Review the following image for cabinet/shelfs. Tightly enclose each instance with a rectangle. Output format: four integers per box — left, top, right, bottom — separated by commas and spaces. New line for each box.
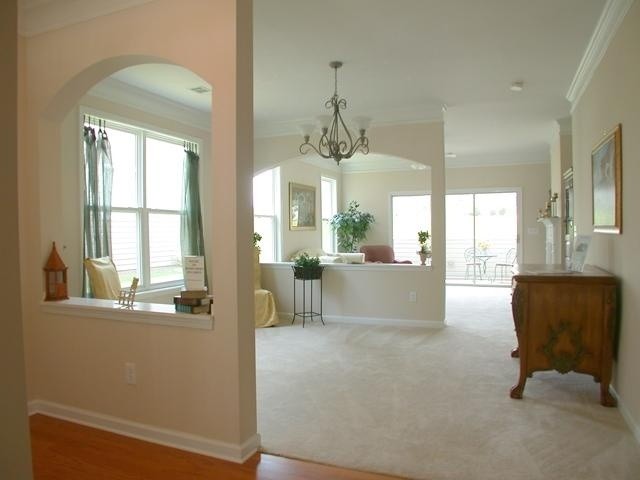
505, 264, 622, 410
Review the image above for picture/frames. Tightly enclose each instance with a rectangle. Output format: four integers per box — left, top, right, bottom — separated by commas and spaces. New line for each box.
287, 180, 317, 231
590, 123, 624, 236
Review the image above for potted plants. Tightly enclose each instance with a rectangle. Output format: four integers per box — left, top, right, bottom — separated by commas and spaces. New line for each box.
415, 230, 431, 265
291, 252, 325, 279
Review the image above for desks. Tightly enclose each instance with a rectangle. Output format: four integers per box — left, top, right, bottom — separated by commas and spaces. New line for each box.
472, 255, 495, 280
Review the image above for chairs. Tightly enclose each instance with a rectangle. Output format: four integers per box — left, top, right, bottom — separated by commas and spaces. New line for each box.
463, 247, 483, 281
361, 244, 413, 264
491, 248, 518, 281
253, 247, 280, 329
85, 254, 123, 300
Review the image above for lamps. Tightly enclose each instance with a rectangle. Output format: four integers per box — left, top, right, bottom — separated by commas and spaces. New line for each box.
297, 57, 370, 167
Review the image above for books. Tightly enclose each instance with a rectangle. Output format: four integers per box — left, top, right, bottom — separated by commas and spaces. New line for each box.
173, 256, 210, 314
